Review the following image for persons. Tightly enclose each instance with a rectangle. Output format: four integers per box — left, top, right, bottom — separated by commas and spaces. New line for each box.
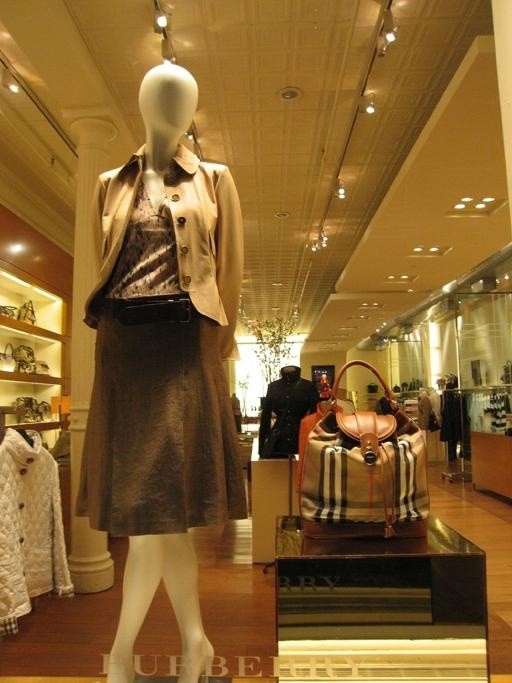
440, 376, 468, 466
259, 365, 320, 457
73, 60, 249, 683
230, 393, 243, 433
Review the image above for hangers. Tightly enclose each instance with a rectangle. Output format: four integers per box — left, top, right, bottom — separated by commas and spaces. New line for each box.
0, 424, 59, 469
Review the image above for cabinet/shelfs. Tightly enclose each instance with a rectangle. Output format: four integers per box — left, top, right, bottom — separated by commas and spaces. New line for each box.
0, 258, 71, 454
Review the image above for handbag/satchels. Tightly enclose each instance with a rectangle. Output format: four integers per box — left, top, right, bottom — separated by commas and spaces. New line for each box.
0, 343, 49, 375
295, 360, 430, 540
18, 300, 36, 325
15, 397, 51, 424
0, 306, 17, 317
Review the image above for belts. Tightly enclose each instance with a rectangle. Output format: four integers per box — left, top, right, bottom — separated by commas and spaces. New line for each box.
113, 294, 197, 326
